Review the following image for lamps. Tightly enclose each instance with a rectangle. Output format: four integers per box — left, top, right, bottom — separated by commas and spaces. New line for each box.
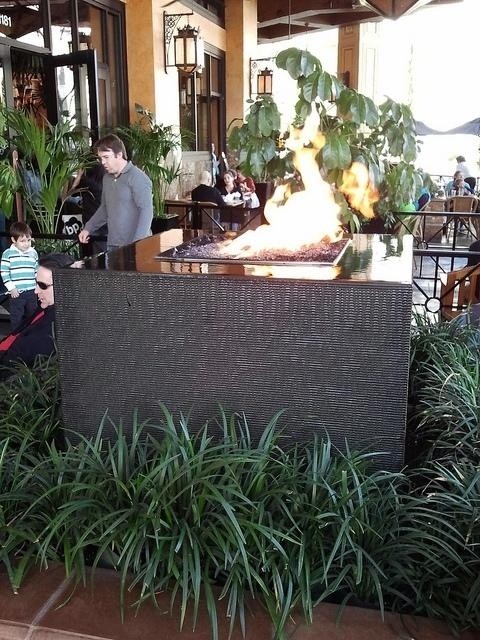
186, 75, 203, 110
249, 58, 277, 98
163, 9, 205, 74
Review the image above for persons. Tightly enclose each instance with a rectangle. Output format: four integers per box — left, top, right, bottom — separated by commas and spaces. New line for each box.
0, 222, 40, 333
187, 165, 260, 230
0, 254, 73, 383
68, 132, 132, 255
77, 135, 153, 269
467, 241, 480, 301
410, 156, 480, 211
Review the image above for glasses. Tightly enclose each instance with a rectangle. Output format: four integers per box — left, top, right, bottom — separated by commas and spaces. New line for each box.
35, 278, 53, 289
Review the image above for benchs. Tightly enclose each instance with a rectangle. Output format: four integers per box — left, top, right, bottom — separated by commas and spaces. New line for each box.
0, 559, 478, 640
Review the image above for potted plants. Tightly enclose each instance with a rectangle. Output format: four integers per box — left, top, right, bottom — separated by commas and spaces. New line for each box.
94, 103, 197, 235
